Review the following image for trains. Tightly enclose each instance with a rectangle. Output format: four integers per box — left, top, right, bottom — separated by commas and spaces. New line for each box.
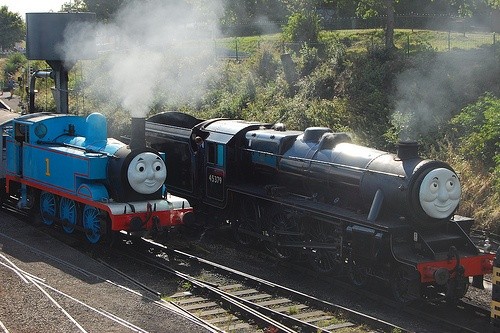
1, 112, 193, 246
118, 111, 494, 306
